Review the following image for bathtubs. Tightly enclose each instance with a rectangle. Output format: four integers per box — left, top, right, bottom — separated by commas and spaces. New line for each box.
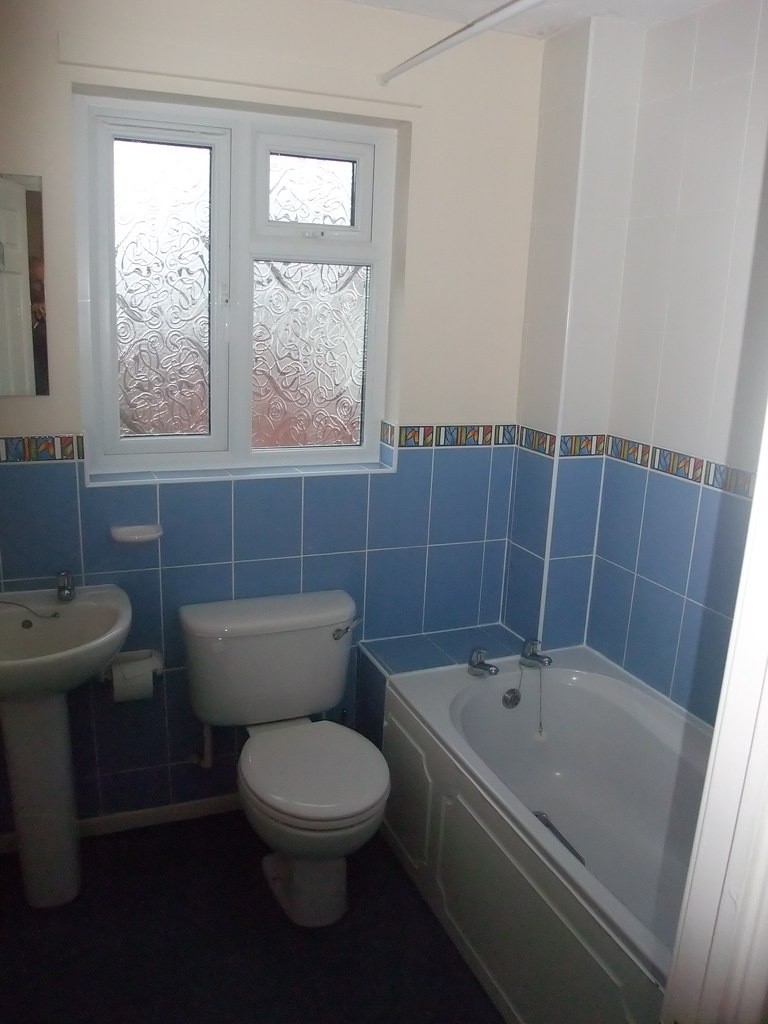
381, 644, 718, 1023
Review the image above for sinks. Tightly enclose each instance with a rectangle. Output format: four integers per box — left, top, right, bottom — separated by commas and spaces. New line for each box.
0, 584, 130, 701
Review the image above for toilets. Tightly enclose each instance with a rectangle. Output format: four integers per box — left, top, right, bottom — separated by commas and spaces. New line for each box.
180, 592, 391, 928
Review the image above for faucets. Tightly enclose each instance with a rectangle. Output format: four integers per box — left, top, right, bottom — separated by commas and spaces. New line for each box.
57, 560, 78, 601
522, 642, 553, 667
469, 648, 499, 678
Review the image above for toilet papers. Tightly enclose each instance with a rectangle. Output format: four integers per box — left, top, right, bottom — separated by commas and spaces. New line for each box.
111, 661, 155, 702
